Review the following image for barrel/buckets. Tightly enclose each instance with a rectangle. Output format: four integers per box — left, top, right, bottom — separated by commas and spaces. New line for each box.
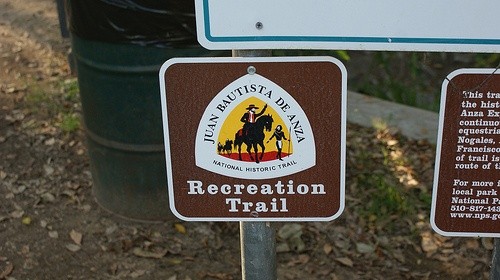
67, 0, 234, 225
